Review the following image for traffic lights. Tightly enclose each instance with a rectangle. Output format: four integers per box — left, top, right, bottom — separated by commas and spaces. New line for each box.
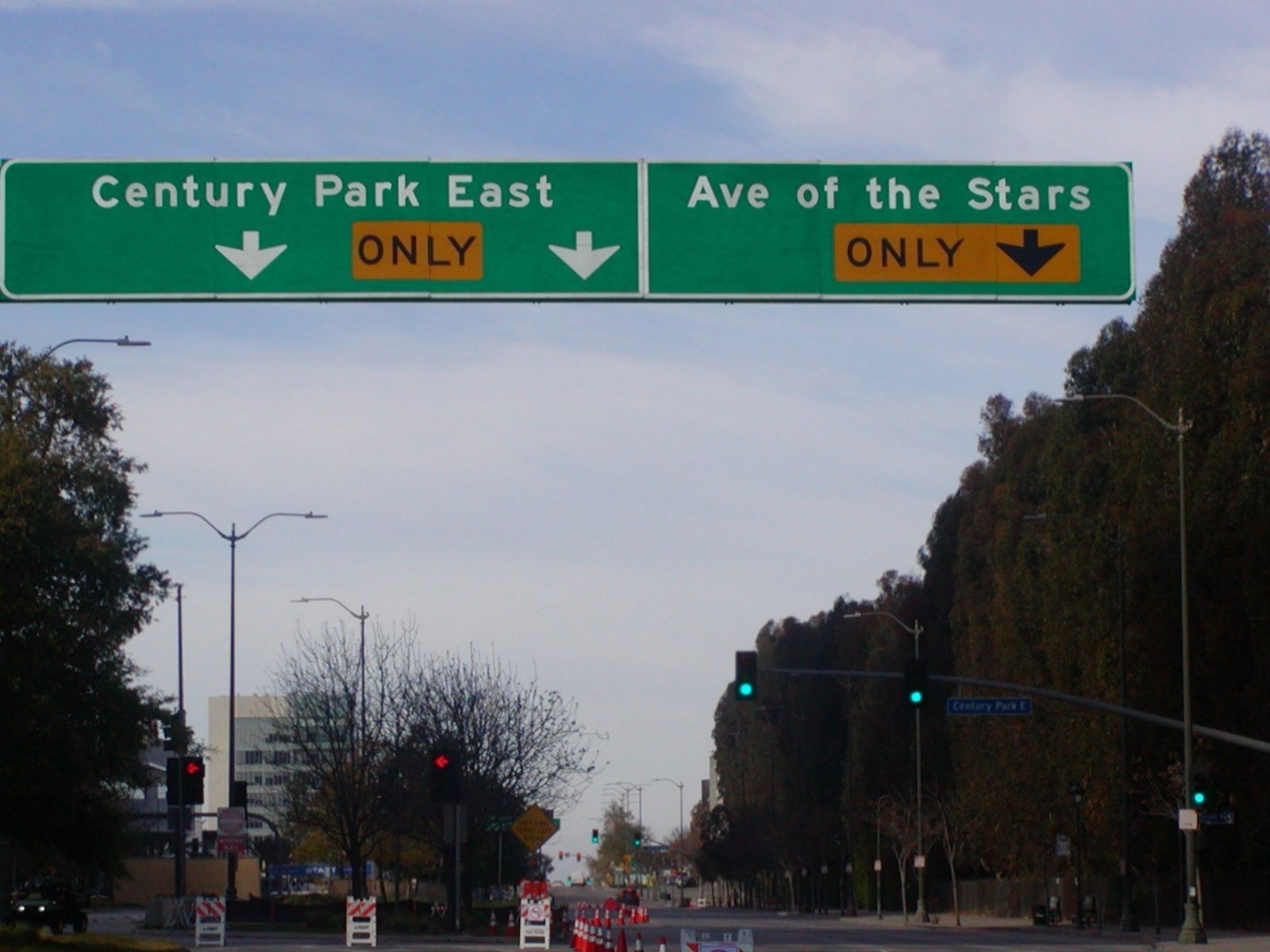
559, 851, 563, 860
592, 829, 600, 843
1190, 780, 1205, 814
167, 753, 206, 804
911, 661, 929, 713
735, 652, 760, 702
578, 853, 582, 861
232, 781, 248, 818
552, 819, 560, 829
634, 832, 643, 846
567, 876, 571, 885
428, 747, 461, 781
161, 708, 188, 751
586, 876, 592, 885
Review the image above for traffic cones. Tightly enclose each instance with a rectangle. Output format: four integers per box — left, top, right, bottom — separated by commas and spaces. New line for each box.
549, 912, 554, 934
508, 912, 518, 938
489, 911, 499, 937
562, 899, 667, 952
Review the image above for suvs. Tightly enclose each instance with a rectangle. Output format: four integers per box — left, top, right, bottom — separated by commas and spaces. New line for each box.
616, 889, 641, 905
675, 875, 699, 888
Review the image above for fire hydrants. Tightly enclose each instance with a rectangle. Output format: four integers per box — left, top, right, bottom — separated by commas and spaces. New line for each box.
931, 914, 940, 924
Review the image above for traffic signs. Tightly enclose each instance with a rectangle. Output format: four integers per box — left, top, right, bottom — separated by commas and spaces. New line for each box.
2, 159, 639, 301
641, 162, 1133, 303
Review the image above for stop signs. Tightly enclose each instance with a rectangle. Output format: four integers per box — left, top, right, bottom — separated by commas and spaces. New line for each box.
219, 807, 246, 836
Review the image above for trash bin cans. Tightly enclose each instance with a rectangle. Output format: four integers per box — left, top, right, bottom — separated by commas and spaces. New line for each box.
1031, 905, 1046, 925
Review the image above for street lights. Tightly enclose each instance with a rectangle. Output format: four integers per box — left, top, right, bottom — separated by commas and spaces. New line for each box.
848, 611, 930, 922
300, 597, 370, 896
1054, 390, 1207, 944
139, 509, 329, 923
592, 782, 643, 895
650, 778, 684, 906
